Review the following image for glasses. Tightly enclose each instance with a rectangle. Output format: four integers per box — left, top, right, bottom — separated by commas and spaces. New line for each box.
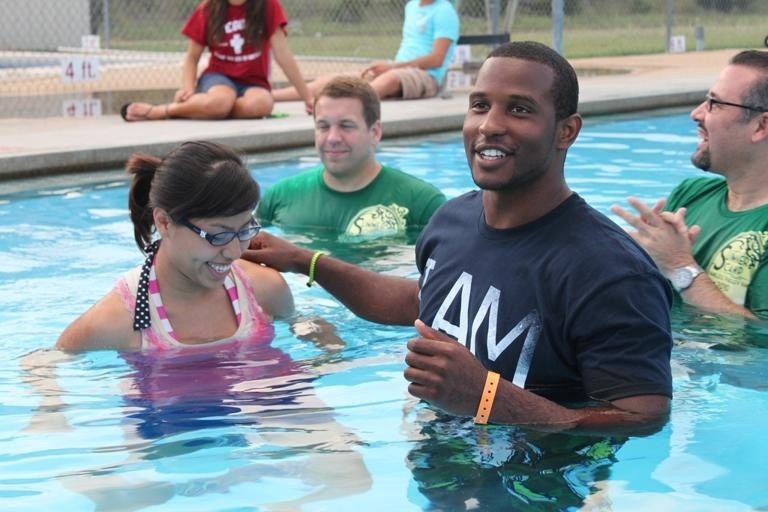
172, 217, 261, 248
704, 95, 759, 116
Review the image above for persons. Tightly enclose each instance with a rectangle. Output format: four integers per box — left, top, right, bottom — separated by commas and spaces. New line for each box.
608, 49, 767, 318
21, 140, 370, 510
251, 76, 448, 237
120, 0, 315, 120
243, 40, 673, 436
270, 0, 460, 105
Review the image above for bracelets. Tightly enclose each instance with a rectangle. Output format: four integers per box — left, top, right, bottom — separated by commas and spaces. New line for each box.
305, 251, 326, 287
472, 371, 502, 427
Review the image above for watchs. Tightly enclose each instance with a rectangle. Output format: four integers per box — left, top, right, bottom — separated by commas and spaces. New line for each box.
671, 263, 705, 293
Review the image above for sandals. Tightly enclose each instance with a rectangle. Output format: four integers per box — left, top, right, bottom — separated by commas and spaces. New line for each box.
120, 102, 170, 121
